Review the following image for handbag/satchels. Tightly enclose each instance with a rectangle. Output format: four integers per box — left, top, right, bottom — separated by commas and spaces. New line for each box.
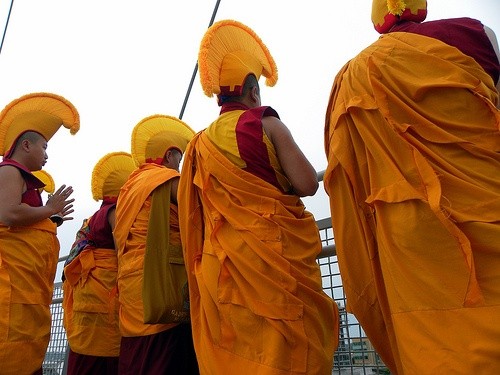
143, 180, 191, 325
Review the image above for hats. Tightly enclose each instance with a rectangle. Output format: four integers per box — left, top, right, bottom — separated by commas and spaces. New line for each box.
0, 92, 80, 159
91, 151, 139, 202
198, 19, 278, 97
31, 170, 55, 194
131, 114, 197, 167
370, 0, 427, 33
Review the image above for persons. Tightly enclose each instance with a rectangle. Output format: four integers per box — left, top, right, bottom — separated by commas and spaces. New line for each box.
324, 0, 500, 375
0, 92, 81, 375
60, 19, 339, 375
